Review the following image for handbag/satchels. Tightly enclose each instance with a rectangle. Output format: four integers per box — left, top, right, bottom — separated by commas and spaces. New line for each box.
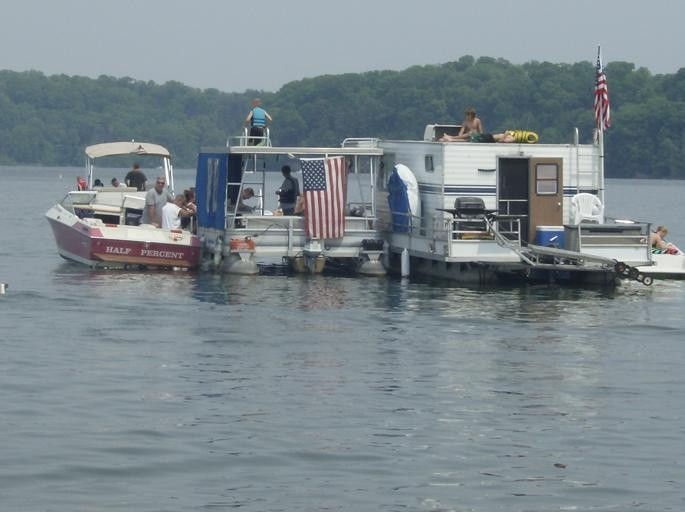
277, 177, 298, 204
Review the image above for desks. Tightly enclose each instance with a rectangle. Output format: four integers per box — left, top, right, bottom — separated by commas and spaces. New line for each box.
435, 203, 507, 239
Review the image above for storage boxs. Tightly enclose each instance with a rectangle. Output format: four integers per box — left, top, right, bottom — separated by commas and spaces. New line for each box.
534, 225, 564, 249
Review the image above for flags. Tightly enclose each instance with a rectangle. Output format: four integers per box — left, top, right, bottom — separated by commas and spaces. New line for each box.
594, 56, 611, 132
299, 156, 344, 240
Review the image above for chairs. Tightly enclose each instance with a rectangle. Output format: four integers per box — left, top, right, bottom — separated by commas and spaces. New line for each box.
570, 192, 605, 226
451, 197, 488, 238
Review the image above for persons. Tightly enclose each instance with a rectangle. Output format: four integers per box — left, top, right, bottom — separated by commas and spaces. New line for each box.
245, 98, 272, 146
276, 165, 299, 216
458, 109, 482, 141
77, 163, 197, 232
238, 187, 255, 212
442, 132, 518, 143
651, 226, 668, 250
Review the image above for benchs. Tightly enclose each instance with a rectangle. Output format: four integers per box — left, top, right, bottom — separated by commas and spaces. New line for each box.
91, 186, 139, 209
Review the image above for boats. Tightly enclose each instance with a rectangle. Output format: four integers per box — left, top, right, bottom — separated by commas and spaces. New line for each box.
44, 138, 201, 272
